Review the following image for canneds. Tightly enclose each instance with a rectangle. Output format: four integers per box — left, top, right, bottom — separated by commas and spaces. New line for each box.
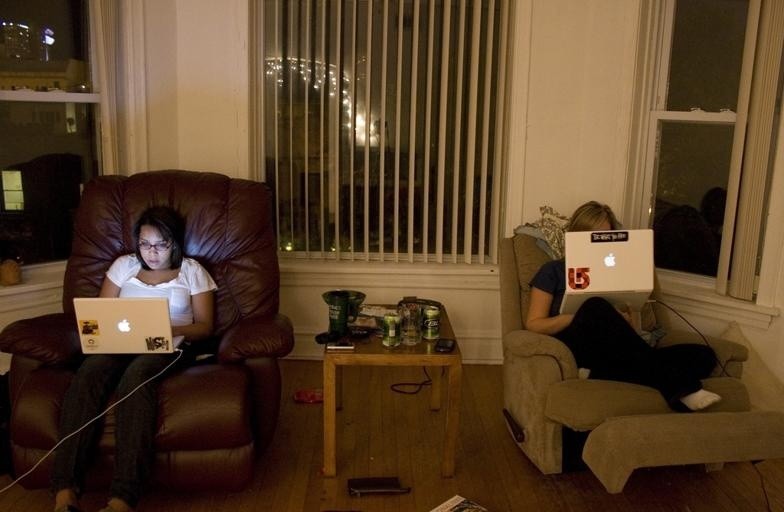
423, 306, 440, 339
382, 312, 401, 347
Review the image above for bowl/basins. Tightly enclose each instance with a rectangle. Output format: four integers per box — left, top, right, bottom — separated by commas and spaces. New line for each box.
323, 290, 366, 306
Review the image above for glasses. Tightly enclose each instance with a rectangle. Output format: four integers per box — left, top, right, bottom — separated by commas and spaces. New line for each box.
138, 240, 172, 251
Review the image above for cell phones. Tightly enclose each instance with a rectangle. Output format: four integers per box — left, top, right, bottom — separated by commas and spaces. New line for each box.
435, 338, 457, 353
326, 341, 356, 349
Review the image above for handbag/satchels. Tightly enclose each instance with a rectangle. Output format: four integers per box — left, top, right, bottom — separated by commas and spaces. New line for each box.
349, 477, 411, 497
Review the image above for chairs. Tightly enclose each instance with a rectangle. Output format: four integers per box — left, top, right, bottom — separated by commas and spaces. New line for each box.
498, 233, 783, 489
1, 169, 295, 489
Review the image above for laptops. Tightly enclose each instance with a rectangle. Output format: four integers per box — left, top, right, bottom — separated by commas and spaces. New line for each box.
560, 230, 656, 314
71, 297, 184, 357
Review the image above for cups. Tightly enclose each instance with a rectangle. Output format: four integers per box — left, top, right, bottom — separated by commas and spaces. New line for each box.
398, 303, 422, 345
328, 291, 357, 333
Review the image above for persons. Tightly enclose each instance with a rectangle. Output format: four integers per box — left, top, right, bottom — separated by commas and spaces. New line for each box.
526, 200, 721, 413
51, 204, 218, 511
